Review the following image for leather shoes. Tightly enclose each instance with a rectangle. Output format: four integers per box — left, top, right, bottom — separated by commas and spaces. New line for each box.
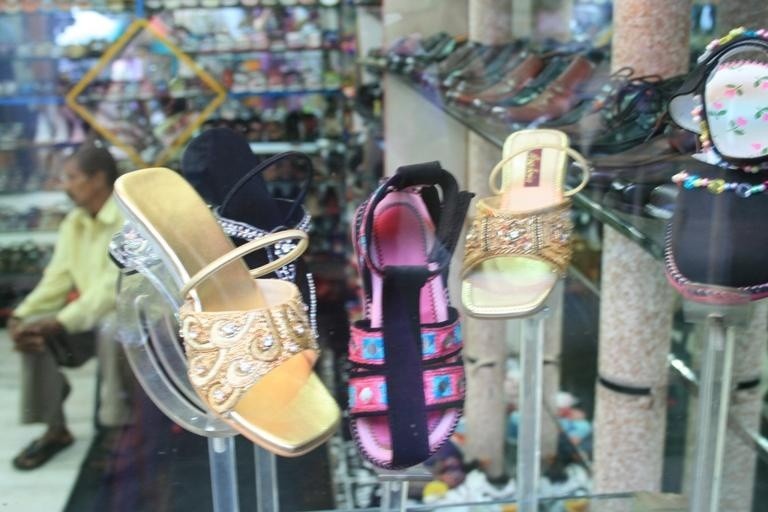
473, 37, 707, 221
365, 29, 480, 106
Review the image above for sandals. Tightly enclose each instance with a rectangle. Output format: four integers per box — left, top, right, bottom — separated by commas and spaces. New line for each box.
181, 127, 319, 336
460, 129, 590, 319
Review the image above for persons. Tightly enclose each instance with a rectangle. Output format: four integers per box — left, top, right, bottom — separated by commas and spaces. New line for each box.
5, 142, 180, 469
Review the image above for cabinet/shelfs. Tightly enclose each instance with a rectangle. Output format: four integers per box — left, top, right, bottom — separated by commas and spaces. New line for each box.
0, 0, 359, 329
385, 32, 768, 512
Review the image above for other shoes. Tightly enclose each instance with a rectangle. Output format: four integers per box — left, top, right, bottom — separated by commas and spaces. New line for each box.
0, 0, 360, 161
99, 424, 142, 475
664, 28, 768, 305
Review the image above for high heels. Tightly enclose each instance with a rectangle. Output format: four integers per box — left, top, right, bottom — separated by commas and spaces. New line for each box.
107, 166, 345, 456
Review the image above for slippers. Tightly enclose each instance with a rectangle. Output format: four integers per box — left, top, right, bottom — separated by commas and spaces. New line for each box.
13, 434, 76, 471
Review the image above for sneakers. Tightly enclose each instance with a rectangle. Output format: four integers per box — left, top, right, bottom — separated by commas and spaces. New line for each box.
337, 361, 593, 511
